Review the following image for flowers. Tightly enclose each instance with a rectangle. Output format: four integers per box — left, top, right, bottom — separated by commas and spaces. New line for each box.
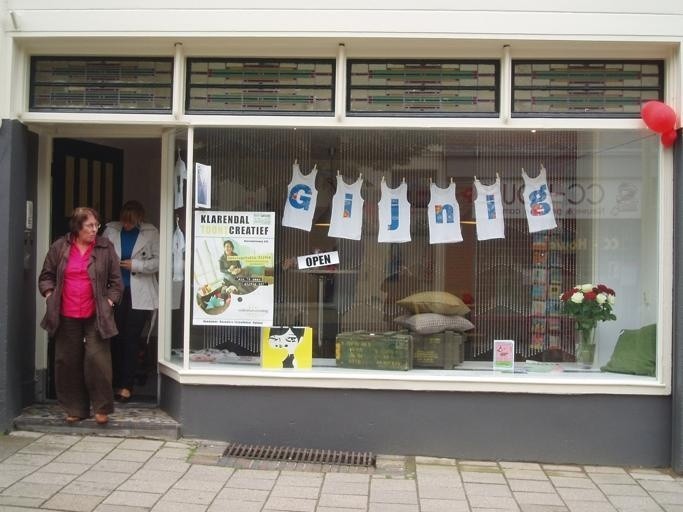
556, 283, 617, 364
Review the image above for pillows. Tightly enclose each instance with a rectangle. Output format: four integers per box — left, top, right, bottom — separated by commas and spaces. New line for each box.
393, 290, 475, 334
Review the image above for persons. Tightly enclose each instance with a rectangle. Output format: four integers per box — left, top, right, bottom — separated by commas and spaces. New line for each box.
219, 239, 241, 286
101, 199, 160, 401
39, 206, 125, 424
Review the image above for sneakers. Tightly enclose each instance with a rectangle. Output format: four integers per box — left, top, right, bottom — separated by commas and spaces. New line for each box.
115, 389, 131, 402
66, 416, 80, 421
96, 414, 107, 423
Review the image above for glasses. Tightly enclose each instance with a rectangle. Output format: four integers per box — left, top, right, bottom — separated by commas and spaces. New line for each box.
86, 223, 101, 228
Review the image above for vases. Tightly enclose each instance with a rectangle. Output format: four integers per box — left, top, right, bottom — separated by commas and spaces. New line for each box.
570, 319, 598, 370
